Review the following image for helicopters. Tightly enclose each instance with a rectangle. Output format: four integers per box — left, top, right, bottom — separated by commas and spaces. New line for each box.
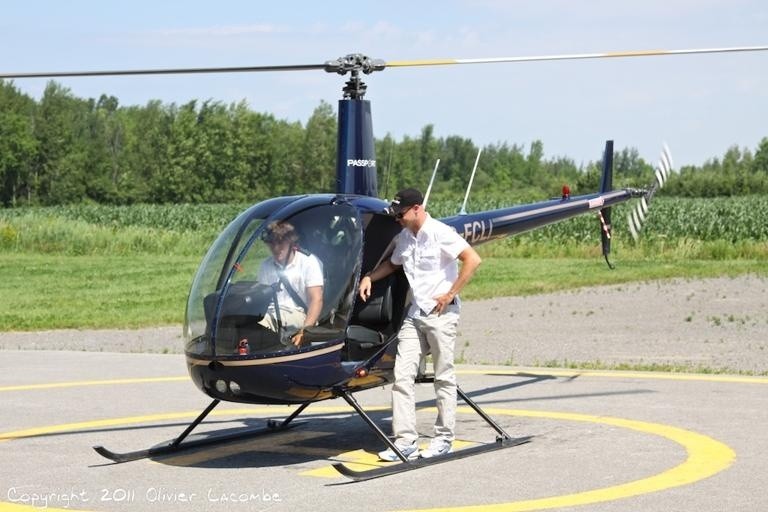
1, 43, 768, 488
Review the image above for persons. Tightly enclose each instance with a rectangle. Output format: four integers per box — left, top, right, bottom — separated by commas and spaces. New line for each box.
359, 189, 482, 462
256, 219, 325, 350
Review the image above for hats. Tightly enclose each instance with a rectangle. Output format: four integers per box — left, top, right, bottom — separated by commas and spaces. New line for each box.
391, 188, 424, 208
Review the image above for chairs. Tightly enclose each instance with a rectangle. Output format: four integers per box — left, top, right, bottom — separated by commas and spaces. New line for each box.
303, 262, 396, 359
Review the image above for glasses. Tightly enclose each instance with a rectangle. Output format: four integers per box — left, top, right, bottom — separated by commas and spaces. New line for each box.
393, 206, 413, 218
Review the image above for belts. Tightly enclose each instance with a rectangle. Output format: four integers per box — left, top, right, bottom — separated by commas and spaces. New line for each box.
448, 299, 456, 305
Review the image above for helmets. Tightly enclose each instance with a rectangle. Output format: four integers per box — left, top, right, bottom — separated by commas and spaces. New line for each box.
261, 219, 300, 242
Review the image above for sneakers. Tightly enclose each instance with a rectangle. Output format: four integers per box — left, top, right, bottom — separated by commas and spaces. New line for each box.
378, 439, 456, 462
